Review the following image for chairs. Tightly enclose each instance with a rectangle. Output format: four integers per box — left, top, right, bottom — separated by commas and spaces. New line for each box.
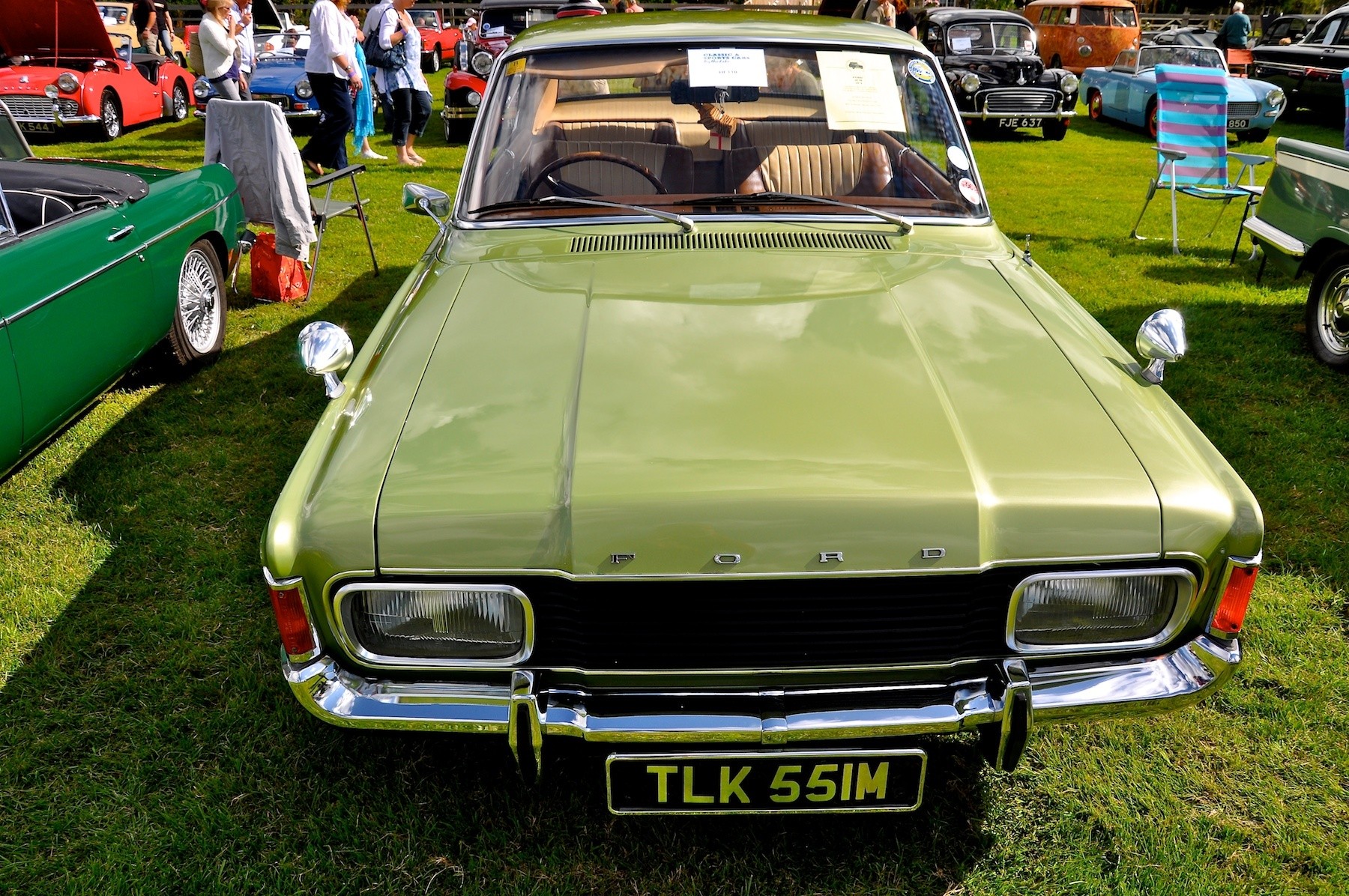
541, 121, 674, 145
731, 142, 896, 198
529, 140, 694, 194
1128, 63, 1272, 261
732, 120, 861, 144
203, 97, 380, 304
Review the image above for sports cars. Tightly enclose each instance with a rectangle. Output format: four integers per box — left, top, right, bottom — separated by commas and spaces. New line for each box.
189, 0, 385, 129
1077, 45, 1287, 142
0, 0, 198, 142
0, 94, 251, 490
403, 9, 468, 73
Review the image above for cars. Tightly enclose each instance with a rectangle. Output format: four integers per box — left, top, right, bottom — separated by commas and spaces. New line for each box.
1249, 3, 1349, 129
1139, 25, 1227, 69
439, 0, 610, 143
901, 9, 1082, 141
91, 2, 189, 72
1249, 14, 1326, 76
258, 10, 1269, 830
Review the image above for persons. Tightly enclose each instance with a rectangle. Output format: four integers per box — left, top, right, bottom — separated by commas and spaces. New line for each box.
1218, 2, 1252, 73
611, 0, 645, 14
416, 17, 427, 26
282, 28, 308, 56
466, 17, 477, 31
133, 0, 175, 61
852, 0, 917, 40
760, 55, 821, 96
556, 0, 610, 101
198, 0, 257, 101
297, 0, 433, 176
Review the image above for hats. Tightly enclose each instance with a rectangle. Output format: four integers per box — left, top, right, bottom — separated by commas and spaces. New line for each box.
466, 16, 478, 26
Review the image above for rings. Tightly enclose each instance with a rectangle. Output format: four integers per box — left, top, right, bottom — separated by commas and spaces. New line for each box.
356, 84, 359, 87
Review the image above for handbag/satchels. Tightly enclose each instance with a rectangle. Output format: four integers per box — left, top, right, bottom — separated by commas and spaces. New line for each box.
362, 6, 413, 70
253, 231, 308, 302
189, 30, 206, 75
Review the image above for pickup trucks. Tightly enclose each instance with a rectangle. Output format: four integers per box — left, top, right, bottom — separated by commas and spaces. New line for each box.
1242, 137, 1349, 371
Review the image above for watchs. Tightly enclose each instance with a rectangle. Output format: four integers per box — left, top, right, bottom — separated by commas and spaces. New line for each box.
345, 66, 351, 70
145, 27, 148, 31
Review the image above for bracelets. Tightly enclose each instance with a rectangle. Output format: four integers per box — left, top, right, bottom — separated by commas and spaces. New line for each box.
400, 29, 407, 36
238, 21, 246, 27
170, 31, 175, 34
347, 70, 356, 78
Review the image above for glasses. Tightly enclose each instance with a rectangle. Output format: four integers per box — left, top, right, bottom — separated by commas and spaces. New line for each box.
225, 6, 230, 10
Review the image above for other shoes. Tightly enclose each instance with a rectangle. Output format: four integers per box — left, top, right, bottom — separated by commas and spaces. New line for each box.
399, 159, 425, 168
301, 156, 324, 176
410, 155, 428, 165
362, 151, 388, 160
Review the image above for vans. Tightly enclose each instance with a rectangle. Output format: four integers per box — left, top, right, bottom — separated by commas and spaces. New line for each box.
1017, 0, 1142, 76
